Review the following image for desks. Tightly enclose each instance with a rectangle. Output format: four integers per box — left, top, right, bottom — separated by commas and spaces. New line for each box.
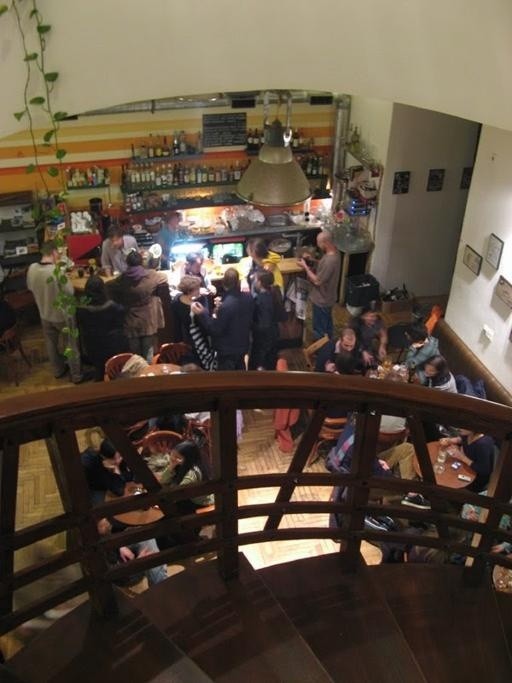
413, 439, 477, 489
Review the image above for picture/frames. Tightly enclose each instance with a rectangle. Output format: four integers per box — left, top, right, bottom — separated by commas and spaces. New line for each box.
485, 232, 505, 271
462, 243, 483, 276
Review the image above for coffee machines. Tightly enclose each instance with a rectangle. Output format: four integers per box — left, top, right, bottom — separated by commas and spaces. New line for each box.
89, 197, 106, 233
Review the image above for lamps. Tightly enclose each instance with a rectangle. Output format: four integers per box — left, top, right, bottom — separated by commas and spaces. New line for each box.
231, 89, 316, 209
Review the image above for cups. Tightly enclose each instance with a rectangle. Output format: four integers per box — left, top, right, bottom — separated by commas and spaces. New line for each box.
70, 211, 95, 234
179, 214, 226, 239
173, 184, 233, 205
369, 355, 408, 383
437, 448, 447, 463
127, 486, 143, 494
161, 194, 170, 206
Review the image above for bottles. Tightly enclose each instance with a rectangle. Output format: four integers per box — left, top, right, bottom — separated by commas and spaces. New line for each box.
122, 159, 252, 187
297, 155, 324, 175
246, 127, 316, 154
67, 165, 110, 187
346, 123, 361, 152
125, 191, 142, 211
324, 219, 353, 251
138, 124, 204, 159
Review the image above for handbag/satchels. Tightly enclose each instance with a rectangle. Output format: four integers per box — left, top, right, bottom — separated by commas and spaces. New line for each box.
189, 324, 212, 370
272, 305, 288, 322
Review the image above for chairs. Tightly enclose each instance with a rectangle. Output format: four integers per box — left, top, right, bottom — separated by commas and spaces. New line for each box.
1, 306, 33, 390
378, 427, 409, 451
307, 417, 350, 467
93, 337, 211, 533
388, 309, 443, 363
327, 467, 370, 543
303, 333, 331, 370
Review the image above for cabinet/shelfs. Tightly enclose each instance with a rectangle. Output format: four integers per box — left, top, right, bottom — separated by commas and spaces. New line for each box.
119, 142, 332, 247
0, 189, 44, 268
